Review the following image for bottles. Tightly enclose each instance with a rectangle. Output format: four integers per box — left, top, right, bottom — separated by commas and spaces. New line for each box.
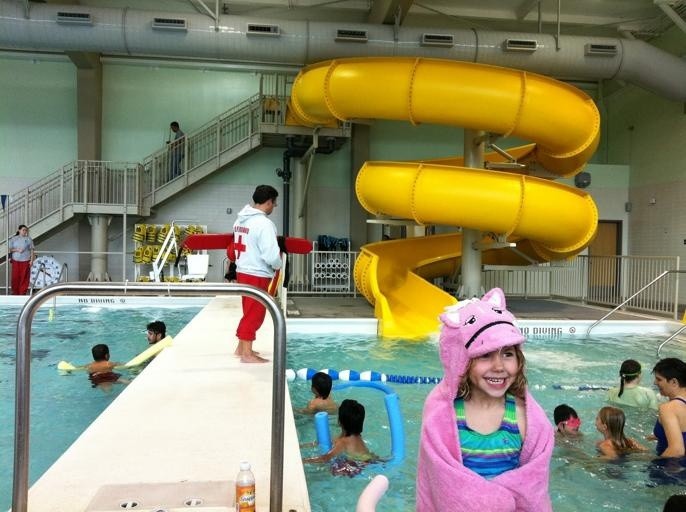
235, 462, 256, 512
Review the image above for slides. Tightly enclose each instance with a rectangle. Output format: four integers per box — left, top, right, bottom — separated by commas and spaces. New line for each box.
287, 56, 601, 340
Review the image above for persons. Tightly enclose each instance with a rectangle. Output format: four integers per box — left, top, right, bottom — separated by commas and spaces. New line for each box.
146, 319, 167, 356
56, 342, 131, 392
299, 397, 380, 465
553, 357, 686, 512
223, 184, 285, 364
291, 371, 341, 416
9, 224, 35, 294
165, 121, 185, 182
415, 286, 556, 512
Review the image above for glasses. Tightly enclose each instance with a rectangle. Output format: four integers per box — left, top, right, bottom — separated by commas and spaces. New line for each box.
558, 418, 580, 427
